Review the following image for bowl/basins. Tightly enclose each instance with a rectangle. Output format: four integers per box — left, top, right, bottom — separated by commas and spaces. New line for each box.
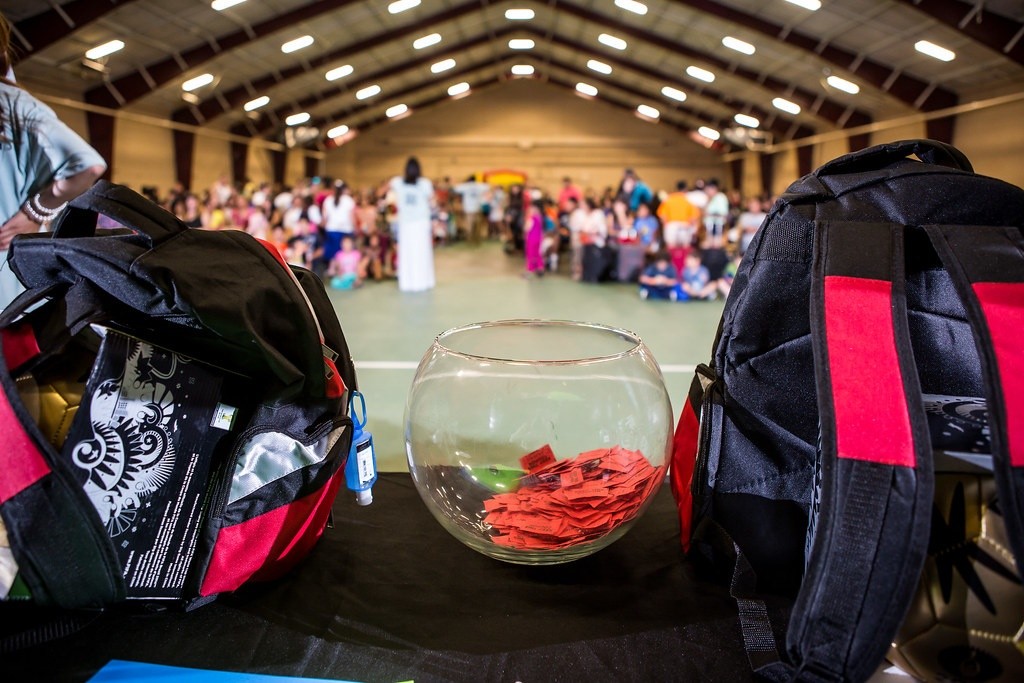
401, 320, 676, 563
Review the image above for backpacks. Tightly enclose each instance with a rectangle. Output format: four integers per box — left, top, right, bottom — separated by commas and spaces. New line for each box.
673, 138, 1024, 683
1, 182, 376, 633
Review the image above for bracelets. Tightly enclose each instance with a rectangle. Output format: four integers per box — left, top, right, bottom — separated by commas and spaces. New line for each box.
23, 188, 69, 224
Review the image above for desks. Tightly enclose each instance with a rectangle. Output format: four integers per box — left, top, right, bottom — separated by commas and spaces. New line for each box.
0, 466, 915, 683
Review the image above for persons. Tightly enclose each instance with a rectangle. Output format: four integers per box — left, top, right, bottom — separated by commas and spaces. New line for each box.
0, 12, 107, 317
138, 155, 779, 305
386, 155, 441, 296
